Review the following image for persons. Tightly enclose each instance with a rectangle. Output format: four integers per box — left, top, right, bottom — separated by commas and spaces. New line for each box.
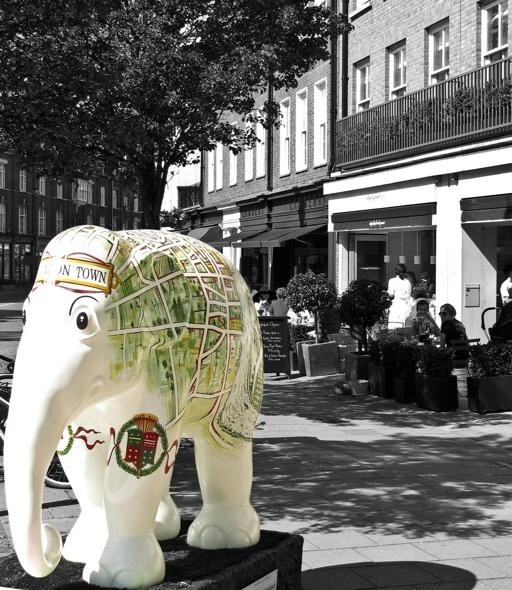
407, 271, 427, 301
433, 303, 471, 376
404, 300, 441, 342
385, 262, 415, 331
499, 275, 512, 305
250, 285, 318, 343
489, 288, 512, 341
419, 271, 434, 299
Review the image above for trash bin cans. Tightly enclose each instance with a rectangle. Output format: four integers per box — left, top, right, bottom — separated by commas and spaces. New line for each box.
259, 316, 291, 379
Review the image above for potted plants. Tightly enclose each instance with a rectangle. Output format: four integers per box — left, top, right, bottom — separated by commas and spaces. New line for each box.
283, 266, 512, 413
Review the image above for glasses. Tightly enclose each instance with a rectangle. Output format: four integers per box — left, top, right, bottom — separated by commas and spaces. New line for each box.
439, 312, 449, 316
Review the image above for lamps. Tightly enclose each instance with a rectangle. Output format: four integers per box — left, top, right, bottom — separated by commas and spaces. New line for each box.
383, 229, 436, 265
